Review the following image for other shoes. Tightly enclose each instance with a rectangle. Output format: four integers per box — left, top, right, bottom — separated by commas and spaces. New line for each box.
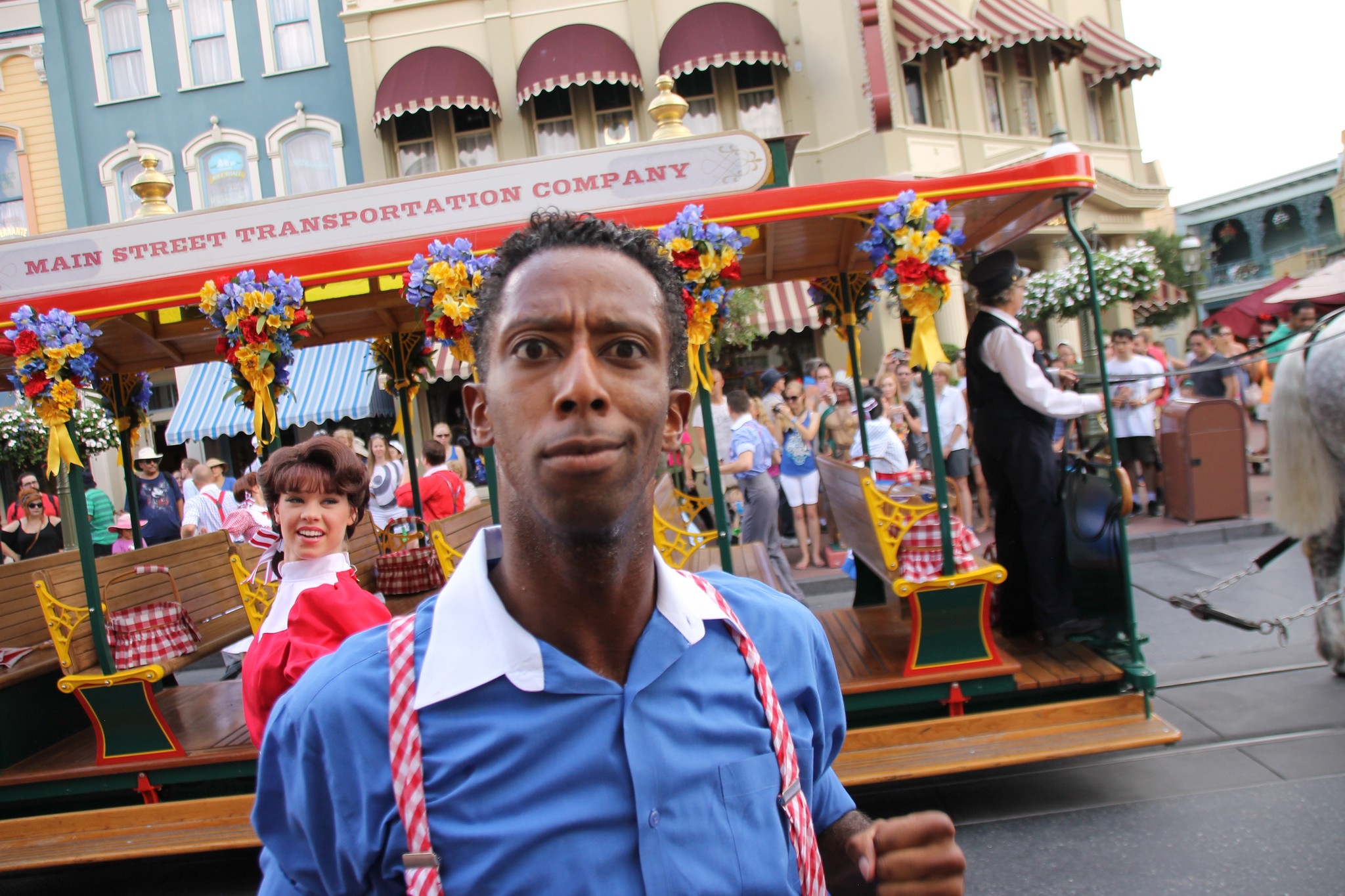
1150, 501, 1163, 517
1129, 505, 1145, 516
782, 538, 811, 547
219, 661, 243, 680
975, 525, 991, 534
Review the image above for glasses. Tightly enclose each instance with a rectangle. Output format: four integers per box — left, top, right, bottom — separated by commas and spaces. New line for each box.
784, 395, 802, 402
28, 503, 43, 509
141, 459, 158, 465
313, 429, 327, 437
434, 434, 451, 439
370, 434, 384, 439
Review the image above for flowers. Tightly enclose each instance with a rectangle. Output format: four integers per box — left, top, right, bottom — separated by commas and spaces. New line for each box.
0, 304, 156, 470
197, 268, 315, 414
358, 234, 501, 398
1014, 240, 1166, 321
806, 192, 969, 337
653, 203, 752, 337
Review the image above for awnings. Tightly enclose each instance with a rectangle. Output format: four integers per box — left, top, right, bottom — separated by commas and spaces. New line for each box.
898, 0, 1162, 93
165, 271, 836, 447
369, 1, 787, 143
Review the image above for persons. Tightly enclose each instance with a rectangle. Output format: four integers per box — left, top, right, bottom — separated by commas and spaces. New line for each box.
960, 247, 1106, 647
0, 301, 1323, 755
250, 203, 967, 896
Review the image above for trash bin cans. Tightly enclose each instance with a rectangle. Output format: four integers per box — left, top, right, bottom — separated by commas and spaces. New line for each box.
1160, 400, 1252, 526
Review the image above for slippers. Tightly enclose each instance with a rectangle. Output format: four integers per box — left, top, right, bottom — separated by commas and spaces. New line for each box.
812, 560, 827, 568
797, 562, 812, 570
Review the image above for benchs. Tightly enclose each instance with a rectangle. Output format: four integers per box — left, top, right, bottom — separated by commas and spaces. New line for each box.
428, 499, 494, 582
32, 526, 252, 769
227, 507, 443, 634
651, 471, 785, 595
814, 452, 1006, 676
0, 548, 178, 771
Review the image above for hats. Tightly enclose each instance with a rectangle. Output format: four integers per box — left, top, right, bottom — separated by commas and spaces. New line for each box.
206, 458, 229, 473
353, 438, 369, 459
134, 447, 164, 471
966, 250, 1030, 291
108, 513, 148, 532
759, 368, 791, 396
388, 441, 404, 455
82, 471, 96, 489
